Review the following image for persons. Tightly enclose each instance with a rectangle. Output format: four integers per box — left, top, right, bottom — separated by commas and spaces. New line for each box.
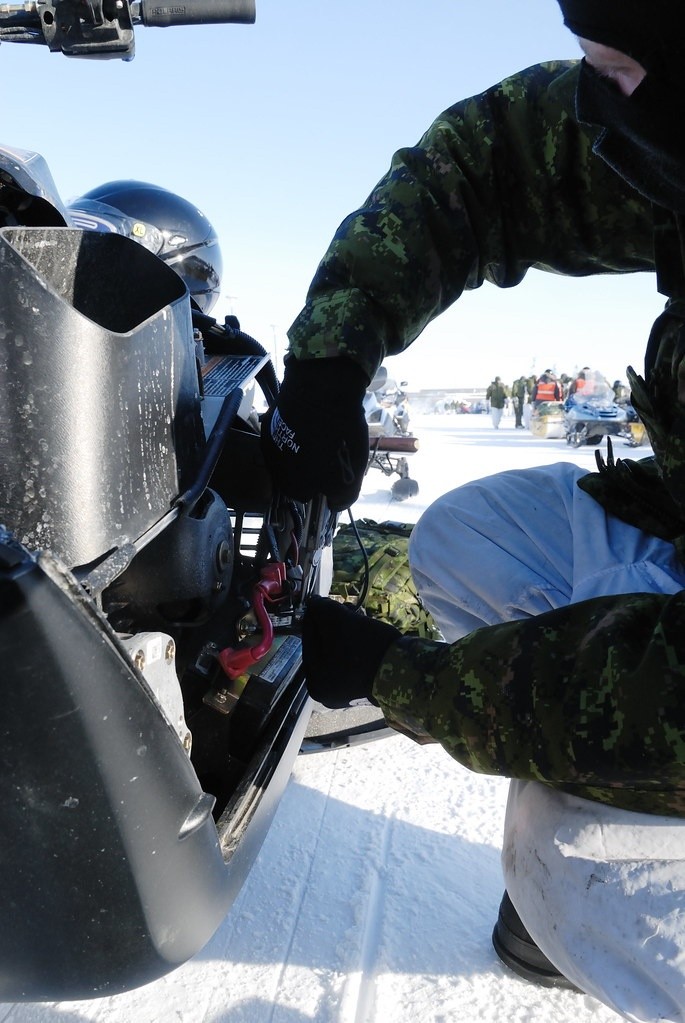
512, 376, 526, 429
526, 367, 629, 409
486, 377, 509, 429
260, 0, 685, 1023
443, 399, 466, 408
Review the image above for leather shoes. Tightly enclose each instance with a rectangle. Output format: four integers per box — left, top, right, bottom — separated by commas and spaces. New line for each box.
491, 890, 584, 997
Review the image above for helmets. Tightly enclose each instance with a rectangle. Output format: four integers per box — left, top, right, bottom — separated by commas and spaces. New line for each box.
64, 179, 223, 314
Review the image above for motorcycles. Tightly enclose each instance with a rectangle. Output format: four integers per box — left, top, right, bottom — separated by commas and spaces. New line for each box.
562, 371, 645, 448
363, 367, 414, 438
1, 0, 335, 1005
524, 375, 567, 438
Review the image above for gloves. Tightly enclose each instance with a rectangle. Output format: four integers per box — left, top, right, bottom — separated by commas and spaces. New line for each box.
294, 590, 384, 703
278, 363, 371, 513
